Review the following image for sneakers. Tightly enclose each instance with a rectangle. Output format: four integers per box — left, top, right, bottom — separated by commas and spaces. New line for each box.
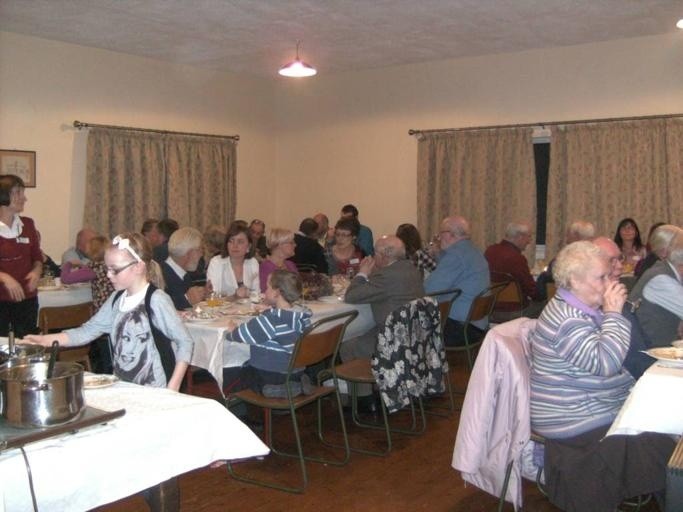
300, 374, 316, 394
264, 380, 301, 399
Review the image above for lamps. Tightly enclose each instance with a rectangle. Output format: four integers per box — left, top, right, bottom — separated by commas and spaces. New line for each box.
675, 17, 683, 29
277, 43, 316, 78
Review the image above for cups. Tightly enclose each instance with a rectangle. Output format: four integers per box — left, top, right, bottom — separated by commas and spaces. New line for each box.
207, 292, 218, 307
607, 286, 642, 314
302, 282, 309, 308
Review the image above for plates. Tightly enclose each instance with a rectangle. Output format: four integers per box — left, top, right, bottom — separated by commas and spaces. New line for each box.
83, 374, 119, 389
672, 339, 683, 346
646, 347, 683, 367
185, 313, 218, 321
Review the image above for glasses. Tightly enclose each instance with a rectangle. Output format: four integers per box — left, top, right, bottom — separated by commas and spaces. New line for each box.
101, 262, 137, 275
335, 233, 353, 238
436, 230, 450, 236
607, 255, 625, 267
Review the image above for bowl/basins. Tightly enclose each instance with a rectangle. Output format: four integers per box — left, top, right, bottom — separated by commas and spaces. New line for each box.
0, 344, 45, 364
0, 363, 84, 428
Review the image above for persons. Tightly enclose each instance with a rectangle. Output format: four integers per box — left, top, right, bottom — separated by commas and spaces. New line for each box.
249, 220, 270, 257
62, 236, 115, 374
331, 205, 375, 256
0, 174, 43, 337
260, 227, 300, 294
536, 220, 595, 302
483, 220, 548, 324
225, 270, 316, 397
338, 234, 426, 426
17, 232, 194, 510
112, 305, 157, 387
159, 227, 213, 310
629, 225, 683, 348
152, 219, 179, 263
592, 237, 658, 380
613, 218, 648, 273
206, 223, 261, 298
36, 230, 61, 277
396, 224, 438, 272
289, 218, 329, 274
61, 227, 100, 275
313, 213, 335, 256
324, 218, 363, 275
423, 215, 490, 345
526, 240, 678, 506
141, 219, 160, 241
634, 222, 670, 276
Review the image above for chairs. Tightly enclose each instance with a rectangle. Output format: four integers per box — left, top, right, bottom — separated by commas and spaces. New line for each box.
316, 289, 463, 457
497, 320, 653, 512
535, 270, 561, 312
488, 271, 542, 323
225, 310, 360, 494
401, 281, 510, 421
38, 303, 92, 375
34, 274, 93, 306
